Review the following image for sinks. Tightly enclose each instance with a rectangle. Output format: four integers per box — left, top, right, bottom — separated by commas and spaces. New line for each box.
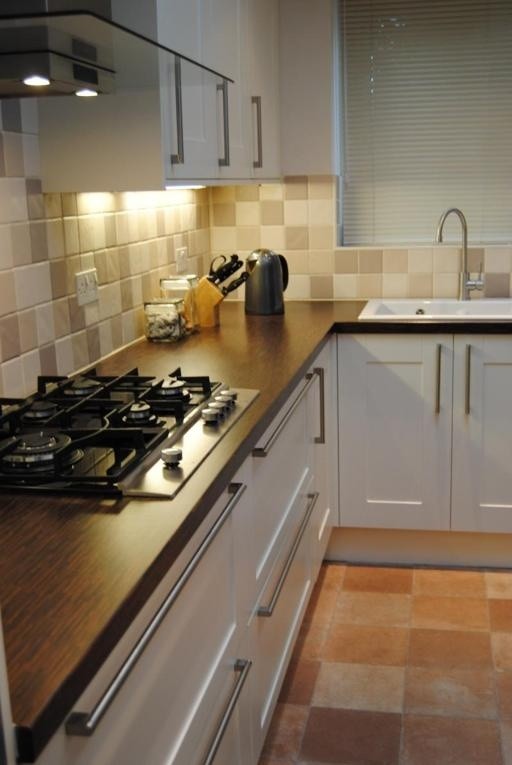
356, 297, 512, 319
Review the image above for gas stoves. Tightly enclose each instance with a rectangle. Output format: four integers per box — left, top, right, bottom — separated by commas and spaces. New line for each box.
0, 362, 258, 501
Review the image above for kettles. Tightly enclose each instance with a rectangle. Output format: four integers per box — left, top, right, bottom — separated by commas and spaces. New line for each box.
244, 246, 290, 316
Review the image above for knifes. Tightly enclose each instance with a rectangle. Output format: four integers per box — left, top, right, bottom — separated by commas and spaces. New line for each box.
209, 254, 249, 297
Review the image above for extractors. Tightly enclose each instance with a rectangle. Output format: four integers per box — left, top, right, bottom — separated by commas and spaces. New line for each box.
0, 0, 118, 100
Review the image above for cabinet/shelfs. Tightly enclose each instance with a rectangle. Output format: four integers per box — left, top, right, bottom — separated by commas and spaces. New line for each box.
43, 2, 284, 194
26, 334, 334, 763
326, 337, 512, 569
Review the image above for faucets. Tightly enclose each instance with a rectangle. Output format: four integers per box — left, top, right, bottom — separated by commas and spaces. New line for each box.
435, 206, 485, 302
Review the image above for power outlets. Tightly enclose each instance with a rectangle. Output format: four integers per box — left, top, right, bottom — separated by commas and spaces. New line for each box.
74, 267, 101, 308
176, 246, 187, 274
